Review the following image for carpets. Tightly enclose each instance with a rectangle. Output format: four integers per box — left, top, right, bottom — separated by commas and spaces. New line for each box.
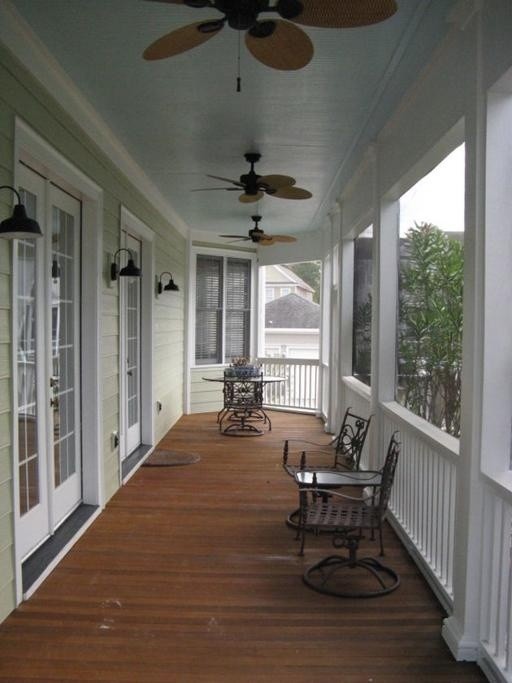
141, 445, 201, 469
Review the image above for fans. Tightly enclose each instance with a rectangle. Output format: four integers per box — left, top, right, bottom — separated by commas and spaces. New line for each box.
139, 1, 402, 71
190, 151, 315, 249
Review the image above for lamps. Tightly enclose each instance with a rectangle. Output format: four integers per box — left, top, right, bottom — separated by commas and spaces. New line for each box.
111, 246, 143, 283
0, 183, 43, 241
157, 270, 180, 294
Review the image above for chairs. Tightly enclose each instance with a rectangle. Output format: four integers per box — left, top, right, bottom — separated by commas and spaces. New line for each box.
281, 406, 405, 600
203, 362, 286, 437
